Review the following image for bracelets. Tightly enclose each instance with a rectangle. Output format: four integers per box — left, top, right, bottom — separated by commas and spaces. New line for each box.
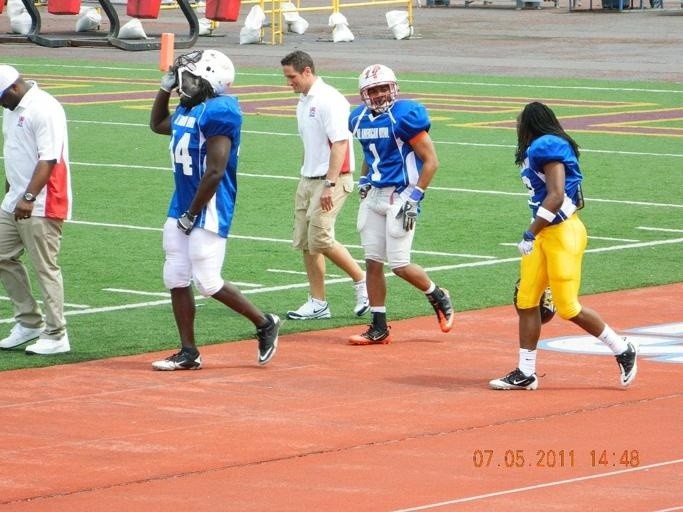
358, 176, 368, 187
409, 186, 424, 204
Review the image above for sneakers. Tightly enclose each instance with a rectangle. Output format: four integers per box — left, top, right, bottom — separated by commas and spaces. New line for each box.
488, 367, 539, 390
614, 337, 640, 385
256, 313, 280, 365
1, 315, 47, 349
426, 286, 454, 332
352, 272, 370, 315
286, 296, 332, 319
152, 350, 201, 370
26, 333, 70, 353
349, 325, 391, 345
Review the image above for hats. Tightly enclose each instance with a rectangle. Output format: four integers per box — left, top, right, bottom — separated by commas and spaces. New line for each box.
0, 65, 20, 99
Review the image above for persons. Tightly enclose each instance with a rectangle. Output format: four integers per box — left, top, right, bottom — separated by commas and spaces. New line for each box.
280, 50, 371, 320
347, 63, 454, 345
489, 102, 637, 390
0, 64, 73, 354
150, 49, 280, 371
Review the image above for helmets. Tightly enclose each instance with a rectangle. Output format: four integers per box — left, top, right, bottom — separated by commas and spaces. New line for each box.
359, 64, 398, 114
514, 278, 556, 324
175, 49, 234, 106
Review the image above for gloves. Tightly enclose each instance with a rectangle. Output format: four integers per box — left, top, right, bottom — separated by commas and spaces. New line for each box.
177, 210, 198, 235
358, 176, 371, 198
518, 231, 535, 254
160, 65, 177, 94
396, 186, 424, 231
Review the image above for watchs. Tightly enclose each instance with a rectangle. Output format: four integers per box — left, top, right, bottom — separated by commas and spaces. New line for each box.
324, 180, 335, 187
24, 193, 36, 202
